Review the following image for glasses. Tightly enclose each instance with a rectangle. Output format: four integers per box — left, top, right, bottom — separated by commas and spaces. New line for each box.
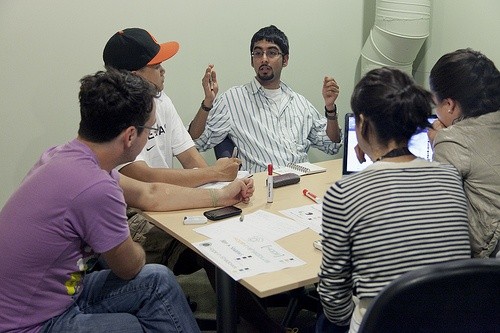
124, 124, 160, 136
251, 51, 284, 58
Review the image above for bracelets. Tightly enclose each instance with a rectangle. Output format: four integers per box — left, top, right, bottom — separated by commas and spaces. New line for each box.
324, 103, 335, 113
202, 100, 213, 111
209, 188, 217, 208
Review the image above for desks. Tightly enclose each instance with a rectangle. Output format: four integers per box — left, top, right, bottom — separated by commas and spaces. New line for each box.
138, 158, 343, 333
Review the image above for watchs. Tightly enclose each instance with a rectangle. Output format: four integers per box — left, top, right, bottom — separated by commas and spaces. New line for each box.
325, 111, 339, 120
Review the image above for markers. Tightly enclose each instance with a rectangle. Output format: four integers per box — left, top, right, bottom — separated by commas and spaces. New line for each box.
267, 164, 274, 203
303, 189, 320, 203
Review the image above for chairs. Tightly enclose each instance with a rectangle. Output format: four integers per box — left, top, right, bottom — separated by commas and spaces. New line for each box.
356, 258, 500, 333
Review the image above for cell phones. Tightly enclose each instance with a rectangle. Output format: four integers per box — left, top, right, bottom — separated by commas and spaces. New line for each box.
203, 204, 242, 221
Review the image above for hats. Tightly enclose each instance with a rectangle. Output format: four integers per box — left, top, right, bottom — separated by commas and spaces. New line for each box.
103, 28, 179, 73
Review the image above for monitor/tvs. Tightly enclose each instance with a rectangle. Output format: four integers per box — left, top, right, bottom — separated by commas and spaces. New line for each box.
342, 113, 439, 175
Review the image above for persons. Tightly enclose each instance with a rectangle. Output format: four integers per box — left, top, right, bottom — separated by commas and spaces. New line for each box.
0, 71, 201, 333
316, 67, 472, 333
187, 25, 344, 174
427, 50, 500, 258
103, 29, 272, 333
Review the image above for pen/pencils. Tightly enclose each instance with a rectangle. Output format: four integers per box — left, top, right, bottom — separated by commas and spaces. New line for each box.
209, 75, 213, 90
232, 146, 238, 158
